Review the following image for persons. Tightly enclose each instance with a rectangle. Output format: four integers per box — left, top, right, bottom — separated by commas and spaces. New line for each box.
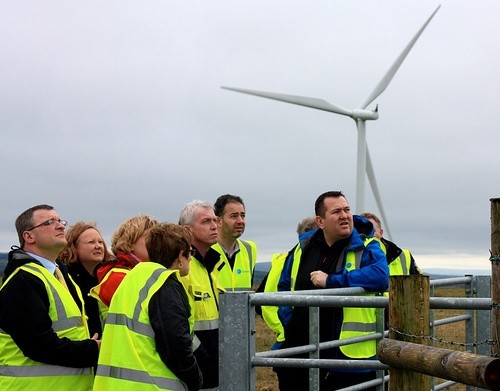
55, 219, 116, 345
89, 215, 162, 338
359, 212, 425, 333
0, 204, 103, 391
178, 199, 235, 391
203, 194, 258, 306
91, 221, 204, 391
273, 190, 390, 391
256, 216, 320, 372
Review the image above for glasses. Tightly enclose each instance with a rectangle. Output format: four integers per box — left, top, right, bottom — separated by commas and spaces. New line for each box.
28, 219, 67, 230
189, 249, 195, 256
